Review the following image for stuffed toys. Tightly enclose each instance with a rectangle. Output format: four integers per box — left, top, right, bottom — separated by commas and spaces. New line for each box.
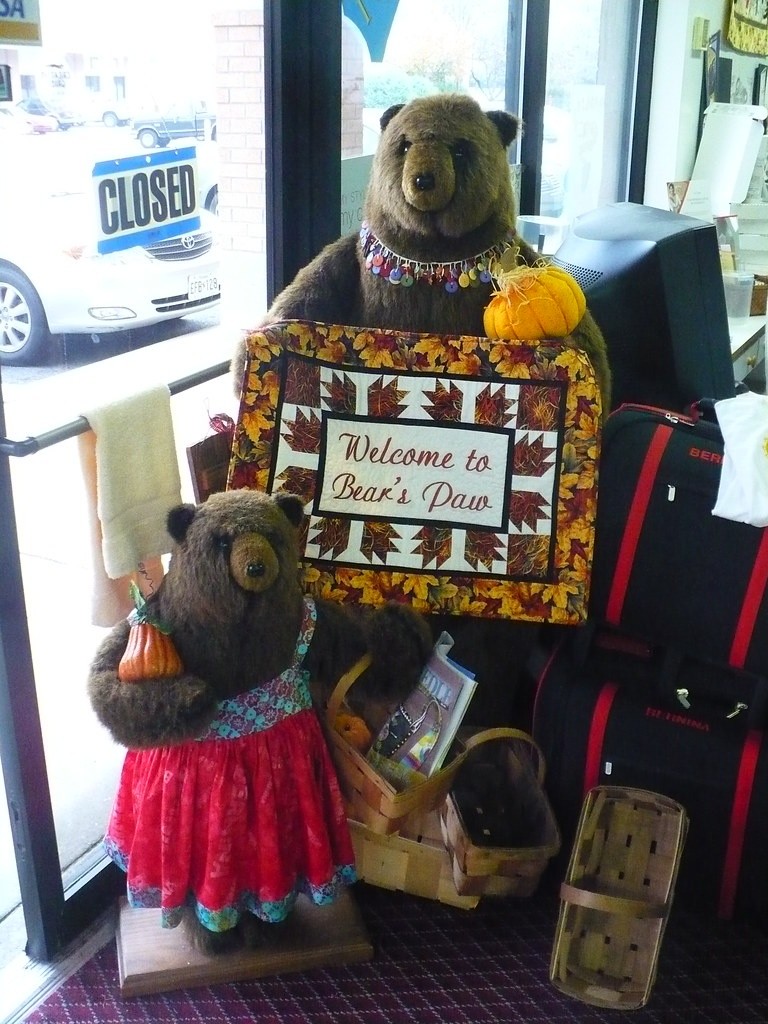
86, 490, 428, 955
232, 93, 608, 711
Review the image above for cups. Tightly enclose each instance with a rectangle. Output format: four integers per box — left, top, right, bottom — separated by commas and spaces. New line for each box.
721, 270, 755, 318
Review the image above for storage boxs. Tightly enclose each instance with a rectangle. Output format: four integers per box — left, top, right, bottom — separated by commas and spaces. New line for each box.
718, 243, 768, 327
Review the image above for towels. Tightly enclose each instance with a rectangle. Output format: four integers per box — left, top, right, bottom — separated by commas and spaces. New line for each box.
77, 381, 184, 629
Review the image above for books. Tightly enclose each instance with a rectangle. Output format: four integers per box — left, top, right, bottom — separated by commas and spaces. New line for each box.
367, 631, 479, 792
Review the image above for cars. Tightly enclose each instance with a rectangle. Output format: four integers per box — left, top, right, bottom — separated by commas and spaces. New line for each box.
0, 76, 222, 362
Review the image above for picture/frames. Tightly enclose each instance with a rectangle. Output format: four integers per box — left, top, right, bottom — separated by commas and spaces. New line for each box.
704, 29, 721, 109
754, 64, 768, 135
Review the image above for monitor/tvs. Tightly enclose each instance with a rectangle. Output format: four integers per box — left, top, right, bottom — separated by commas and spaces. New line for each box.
550, 199, 737, 422
